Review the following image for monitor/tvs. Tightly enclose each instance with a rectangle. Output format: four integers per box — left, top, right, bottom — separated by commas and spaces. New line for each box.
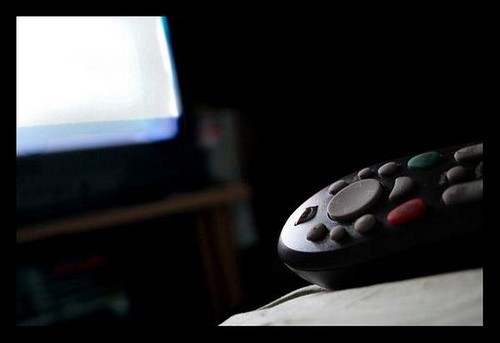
14, 14, 198, 220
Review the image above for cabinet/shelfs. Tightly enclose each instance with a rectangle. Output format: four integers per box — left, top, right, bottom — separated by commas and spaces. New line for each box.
13, 186, 250, 329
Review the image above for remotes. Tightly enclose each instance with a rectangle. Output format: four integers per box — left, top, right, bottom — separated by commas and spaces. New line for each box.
275, 139, 484, 292
